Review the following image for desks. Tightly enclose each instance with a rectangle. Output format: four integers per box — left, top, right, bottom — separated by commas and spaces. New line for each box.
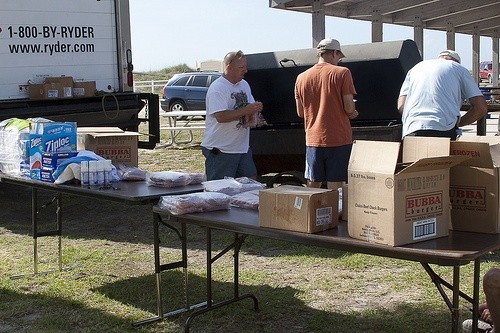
159, 110, 208, 145
0, 171, 215, 332
154, 198, 498, 333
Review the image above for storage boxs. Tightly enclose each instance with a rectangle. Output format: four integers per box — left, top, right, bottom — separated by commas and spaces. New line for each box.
348, 136, 473, 248
29, 75, 97, 99
448, 136, 500, 235
259, 182, 339, 234
19, 120, 141, 183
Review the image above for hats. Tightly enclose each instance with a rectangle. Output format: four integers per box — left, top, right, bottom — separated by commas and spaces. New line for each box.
316, 38, 347, 59
438, 50, 461, 63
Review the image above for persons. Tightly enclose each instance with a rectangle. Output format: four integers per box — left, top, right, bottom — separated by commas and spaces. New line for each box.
295, 38, 359, 188
200, 51, 263, 181
398, 49, 487, 141
478, 268, 500, 333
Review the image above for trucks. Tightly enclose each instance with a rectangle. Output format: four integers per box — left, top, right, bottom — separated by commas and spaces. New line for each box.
0, 0, 162, 152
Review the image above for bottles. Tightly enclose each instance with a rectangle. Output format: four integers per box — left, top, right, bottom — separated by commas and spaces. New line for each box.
80, 161, 89, 185
337, 188, 343, 214
89, 160, 112, 187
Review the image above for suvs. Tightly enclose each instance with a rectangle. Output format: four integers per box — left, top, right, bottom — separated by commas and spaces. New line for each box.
160, 70, 225, 121
480, 60, 500, 80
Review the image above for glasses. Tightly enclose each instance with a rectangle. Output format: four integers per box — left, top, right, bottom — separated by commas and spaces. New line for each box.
229, 50, 244, 64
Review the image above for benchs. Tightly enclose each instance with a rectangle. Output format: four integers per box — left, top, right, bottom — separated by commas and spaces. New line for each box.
160, 123, 206, 143
